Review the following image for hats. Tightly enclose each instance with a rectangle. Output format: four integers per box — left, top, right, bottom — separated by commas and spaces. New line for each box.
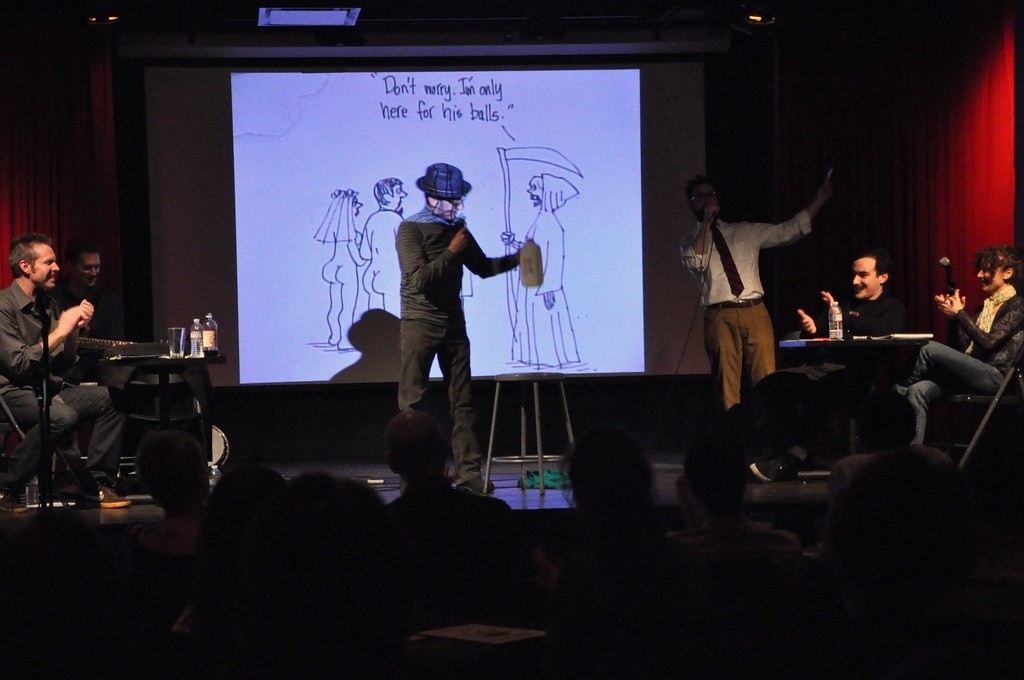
416, 163, 472, 198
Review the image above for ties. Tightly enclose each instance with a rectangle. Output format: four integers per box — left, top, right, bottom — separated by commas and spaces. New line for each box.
710, 222, 744, 298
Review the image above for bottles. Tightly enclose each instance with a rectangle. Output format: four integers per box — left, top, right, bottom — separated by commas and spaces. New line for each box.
208, 464, 221, 493
189, 319, 204, 358
828, 301, 843, 339
201, 313, 218, 351
519, 238, 543, 286
25, 475, 41, 508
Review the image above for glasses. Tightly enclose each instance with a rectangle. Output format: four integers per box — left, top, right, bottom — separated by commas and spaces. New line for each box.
689, 191, 719, 201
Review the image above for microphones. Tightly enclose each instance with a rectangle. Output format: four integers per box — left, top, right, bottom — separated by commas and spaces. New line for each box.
454, 218, 465, 239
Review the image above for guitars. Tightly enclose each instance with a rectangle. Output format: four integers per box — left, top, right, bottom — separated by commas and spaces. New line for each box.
72, 336, 139, 365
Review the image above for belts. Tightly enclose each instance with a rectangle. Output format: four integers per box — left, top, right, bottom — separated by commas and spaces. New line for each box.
704, 298, 763, 310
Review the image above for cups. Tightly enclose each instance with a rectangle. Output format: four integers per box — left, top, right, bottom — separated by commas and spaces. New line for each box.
167, 327, 185, 359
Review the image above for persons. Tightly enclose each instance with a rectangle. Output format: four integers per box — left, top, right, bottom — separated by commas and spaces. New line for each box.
546, 387, 968, 680
0, 410, 520, 680
394, 164, 521, 492
0, 232, 152, 509
680, 175, 1024, 482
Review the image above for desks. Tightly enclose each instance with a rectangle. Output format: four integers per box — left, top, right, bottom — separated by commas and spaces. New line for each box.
780, 332, 929, 480
99, 352, 227, 500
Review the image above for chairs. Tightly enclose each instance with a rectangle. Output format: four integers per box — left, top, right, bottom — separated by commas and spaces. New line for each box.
0, 396, 88, 510
934, 338, 1024, 472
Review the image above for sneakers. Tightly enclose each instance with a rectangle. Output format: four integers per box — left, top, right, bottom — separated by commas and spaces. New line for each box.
0, 488, 28, 512
749, 449, 816, 482
457, 477, 495, 491
75, 485, 131, 509
